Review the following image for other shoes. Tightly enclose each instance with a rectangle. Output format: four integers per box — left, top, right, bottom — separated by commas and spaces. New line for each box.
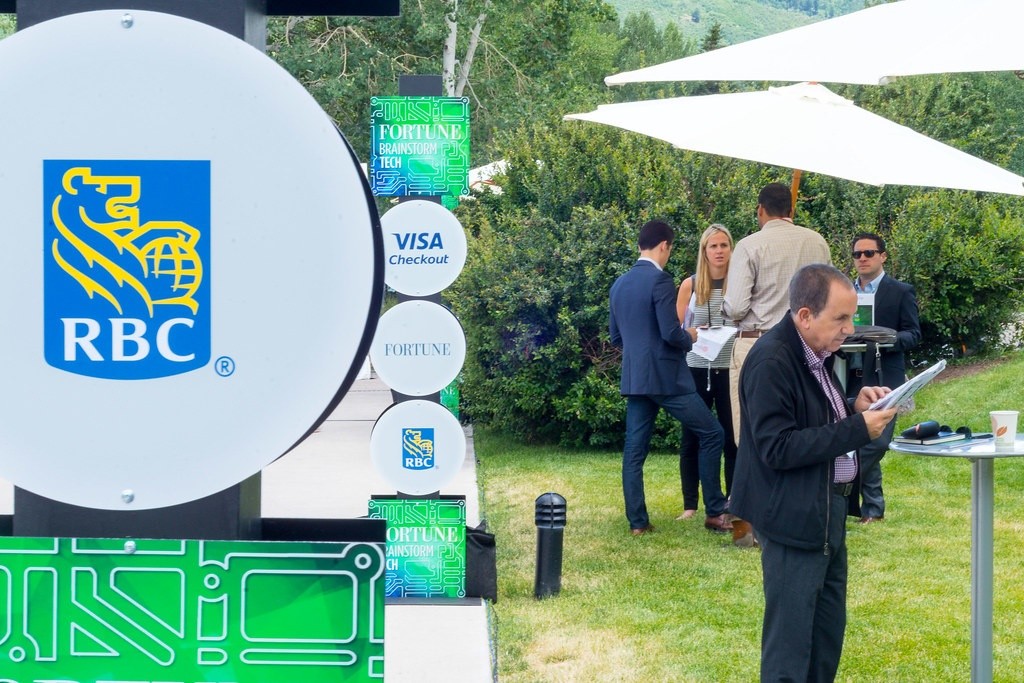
676, 512, 698, 521
858, 517, 881, 524
727, 518, 754, 546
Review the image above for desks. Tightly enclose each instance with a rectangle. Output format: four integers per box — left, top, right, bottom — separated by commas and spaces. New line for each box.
840, 342, 895, 352
889, 434, 1024, 683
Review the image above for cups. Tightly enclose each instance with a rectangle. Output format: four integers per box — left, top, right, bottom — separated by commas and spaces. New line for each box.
990, 410, 1019, 448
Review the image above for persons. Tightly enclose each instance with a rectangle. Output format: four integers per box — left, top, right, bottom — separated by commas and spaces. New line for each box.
675, 223, 738, 521
609, 220, 734, 535
727, 264, 897, 683
845, 232, 920, 525
719, 183, 834, 548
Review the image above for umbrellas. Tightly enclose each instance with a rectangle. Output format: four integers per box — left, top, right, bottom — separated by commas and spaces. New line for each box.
563, 80, 1024, 218
604, 0, 1024, 88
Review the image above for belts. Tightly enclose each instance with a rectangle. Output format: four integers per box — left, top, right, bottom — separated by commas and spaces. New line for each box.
833, 483, 853, 496
735, 331, 765, 338
849, 368, 863, 377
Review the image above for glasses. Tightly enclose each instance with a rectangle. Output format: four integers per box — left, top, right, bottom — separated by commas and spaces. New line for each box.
852, 249, 882, 259
938, 425, 992, 439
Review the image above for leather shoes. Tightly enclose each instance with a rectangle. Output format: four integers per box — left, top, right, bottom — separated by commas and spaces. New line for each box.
632, 523, 655, 536
705, 514, 734, 532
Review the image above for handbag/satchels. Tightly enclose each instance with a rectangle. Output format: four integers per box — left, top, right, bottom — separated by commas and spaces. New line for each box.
842, 325, 898, 345
